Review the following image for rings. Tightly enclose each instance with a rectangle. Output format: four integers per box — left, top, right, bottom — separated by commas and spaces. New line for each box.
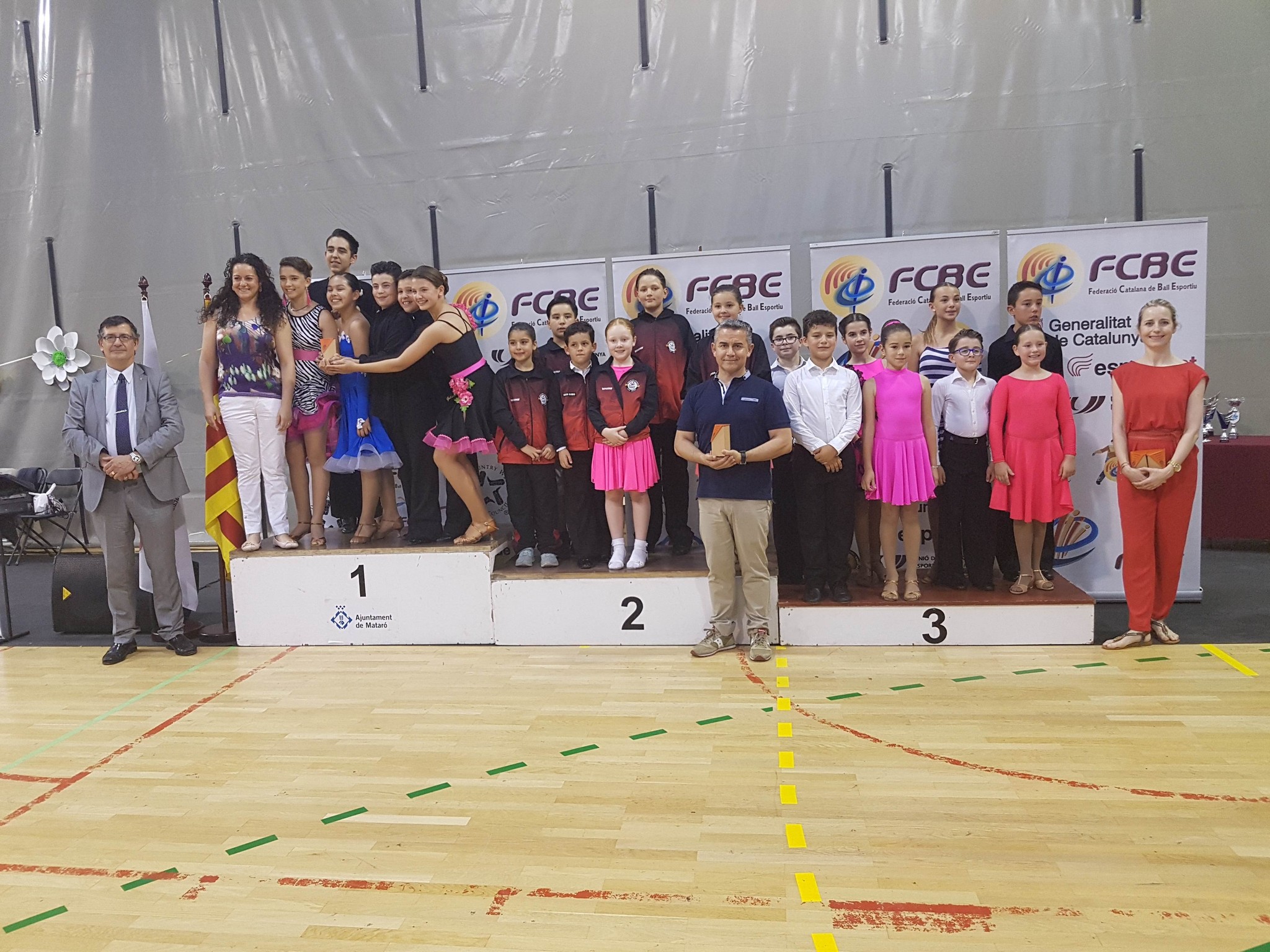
287, 423, 290, 424
111, 471, 115, 475
123, 476, 128, 479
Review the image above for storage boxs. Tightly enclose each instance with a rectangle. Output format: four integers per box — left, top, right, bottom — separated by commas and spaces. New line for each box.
711, 423, 730, 457
1131, 448, 1166, 484
320, 338, 337, 361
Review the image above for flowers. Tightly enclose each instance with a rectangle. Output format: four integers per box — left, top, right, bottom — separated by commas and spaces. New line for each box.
32, 326, 91, 392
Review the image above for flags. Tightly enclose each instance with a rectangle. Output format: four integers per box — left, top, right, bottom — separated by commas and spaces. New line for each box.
204, 295, 247, 583
139, 299, 197, 612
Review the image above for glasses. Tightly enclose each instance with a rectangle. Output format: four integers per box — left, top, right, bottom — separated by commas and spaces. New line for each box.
952, 347, 983, 357
102, 334, 132, 342
771, 336, 800, 346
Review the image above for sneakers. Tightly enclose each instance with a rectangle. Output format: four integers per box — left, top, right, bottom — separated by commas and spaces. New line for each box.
515, 547, 535, 566
540, 553, 559, 567
690, 628, 736, 656
748, 627, 772, 662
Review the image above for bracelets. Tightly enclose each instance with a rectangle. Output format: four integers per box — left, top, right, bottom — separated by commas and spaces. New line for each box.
1119, 463, 1130, 474
932, 466, 938, 469
740, 451, 746, 466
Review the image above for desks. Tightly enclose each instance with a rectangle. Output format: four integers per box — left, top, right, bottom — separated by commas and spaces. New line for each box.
1203, 435, 1270, 552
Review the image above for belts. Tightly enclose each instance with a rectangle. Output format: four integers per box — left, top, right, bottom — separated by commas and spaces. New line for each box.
944, 431, 986, 444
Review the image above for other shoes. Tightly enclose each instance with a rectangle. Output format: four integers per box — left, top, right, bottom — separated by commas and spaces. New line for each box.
575, 529, 694, 569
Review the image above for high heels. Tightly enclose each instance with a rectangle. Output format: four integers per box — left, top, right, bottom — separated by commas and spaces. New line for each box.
371, 516, 404, 541
350, 523, 376, 543
310, 520, 326, 546
289, 522, 311, 541
457, 518, 499, 545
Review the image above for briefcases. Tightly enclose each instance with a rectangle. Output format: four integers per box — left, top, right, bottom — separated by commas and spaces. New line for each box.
0, 474, 35, 513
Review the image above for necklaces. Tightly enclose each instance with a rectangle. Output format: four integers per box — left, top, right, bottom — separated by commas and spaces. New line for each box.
290, 303, 308, 311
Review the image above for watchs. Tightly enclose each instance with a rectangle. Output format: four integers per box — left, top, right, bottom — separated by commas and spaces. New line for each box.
1167, 460, 1181, 472
129, 452, 141, 465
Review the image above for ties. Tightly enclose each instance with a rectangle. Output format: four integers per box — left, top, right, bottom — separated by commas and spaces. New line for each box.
115, 373, 133, 455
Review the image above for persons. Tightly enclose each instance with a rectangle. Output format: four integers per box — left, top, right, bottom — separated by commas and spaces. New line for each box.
279, 217, 1209, 600
199, 253, 299, 550
60, 316, 197, 666
1101, 299, 1209, 650
673, 321, 792, 662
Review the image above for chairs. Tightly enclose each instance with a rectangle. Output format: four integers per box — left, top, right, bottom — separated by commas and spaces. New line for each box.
0, 468, 92, 565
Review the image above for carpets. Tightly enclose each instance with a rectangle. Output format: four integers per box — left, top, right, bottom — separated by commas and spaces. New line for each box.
0, 555, 238, 646
1094, 549, 1270, 646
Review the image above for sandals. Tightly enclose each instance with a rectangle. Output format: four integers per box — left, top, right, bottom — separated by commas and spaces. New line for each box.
1150, 620, 1180, 644
1104, 630, 1154, 649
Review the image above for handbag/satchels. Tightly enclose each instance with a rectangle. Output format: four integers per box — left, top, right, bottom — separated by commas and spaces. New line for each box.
27, 482, 56, 515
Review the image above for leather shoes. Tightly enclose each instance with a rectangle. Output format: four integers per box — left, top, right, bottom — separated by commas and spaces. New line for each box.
409, 538, 424, 544
342, 524, 354, 532
166, 634, 198, 656
273, 536, 299, 548
436, 537, 452, 542
783, 555, 1054, 603
102, 638, 137, 665
241, 538, 261, 550
